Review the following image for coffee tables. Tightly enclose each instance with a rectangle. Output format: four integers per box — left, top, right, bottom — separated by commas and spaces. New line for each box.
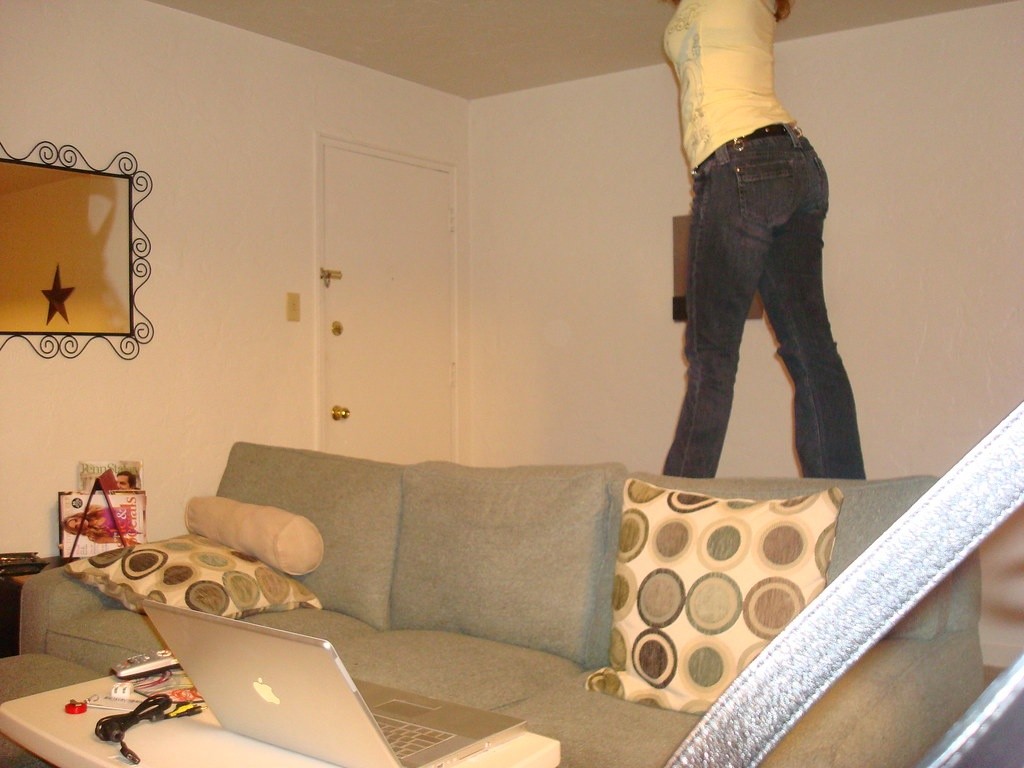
0, 652, 562, 768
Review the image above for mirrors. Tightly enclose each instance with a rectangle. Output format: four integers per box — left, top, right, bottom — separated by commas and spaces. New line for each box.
0, 140, 154, 360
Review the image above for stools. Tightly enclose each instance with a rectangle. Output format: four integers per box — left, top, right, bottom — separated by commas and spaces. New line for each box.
0, 653, 106, 768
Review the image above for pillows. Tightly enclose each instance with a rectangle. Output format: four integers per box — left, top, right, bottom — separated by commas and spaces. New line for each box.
583, 477, 845, 715
62, 534, 323, 620
184, 496, 325, 577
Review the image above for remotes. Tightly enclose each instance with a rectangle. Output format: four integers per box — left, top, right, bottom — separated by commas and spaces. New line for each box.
111, 649, 184, 680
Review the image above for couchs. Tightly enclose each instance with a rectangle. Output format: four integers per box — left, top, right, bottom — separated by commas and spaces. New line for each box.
18, 442, 986, 768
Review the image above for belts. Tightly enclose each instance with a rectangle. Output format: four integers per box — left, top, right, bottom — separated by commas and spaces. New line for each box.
694, 124, 787, 171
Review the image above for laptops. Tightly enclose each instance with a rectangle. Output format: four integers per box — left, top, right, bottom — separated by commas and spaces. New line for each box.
143, 597, 529, 768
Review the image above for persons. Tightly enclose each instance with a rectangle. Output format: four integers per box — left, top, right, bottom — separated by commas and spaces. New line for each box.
116, 471, 137, 490
661, 0, 867, 479
64, 505, 142, 546
